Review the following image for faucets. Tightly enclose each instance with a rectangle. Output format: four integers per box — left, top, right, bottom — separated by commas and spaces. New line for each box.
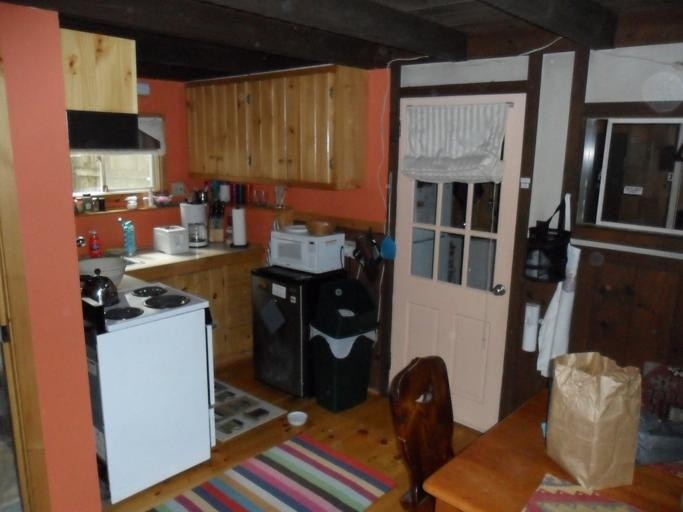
77, 235, 86, 248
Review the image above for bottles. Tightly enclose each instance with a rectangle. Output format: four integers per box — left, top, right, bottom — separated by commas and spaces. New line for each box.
72, 194, 104, 214
118, 219, 136, 256
204, 180, 251, 202
88, 232, 101, 258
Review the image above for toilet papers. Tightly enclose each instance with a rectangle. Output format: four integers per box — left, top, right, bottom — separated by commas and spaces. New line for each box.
232, 207, 247, 245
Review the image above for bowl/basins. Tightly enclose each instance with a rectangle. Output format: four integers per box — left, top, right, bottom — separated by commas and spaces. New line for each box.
287, 410, 306, 426
307, 221, 337, 236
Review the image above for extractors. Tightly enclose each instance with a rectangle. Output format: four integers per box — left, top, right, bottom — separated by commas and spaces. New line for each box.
65, 110, 161, 156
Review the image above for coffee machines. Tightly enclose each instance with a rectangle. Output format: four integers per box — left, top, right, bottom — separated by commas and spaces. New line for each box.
179, 202, 207, 246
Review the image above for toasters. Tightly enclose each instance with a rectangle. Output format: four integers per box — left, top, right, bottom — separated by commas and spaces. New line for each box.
152, 225, 188, 254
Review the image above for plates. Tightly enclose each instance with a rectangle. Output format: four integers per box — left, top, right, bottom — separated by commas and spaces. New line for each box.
286, 224, 306, 232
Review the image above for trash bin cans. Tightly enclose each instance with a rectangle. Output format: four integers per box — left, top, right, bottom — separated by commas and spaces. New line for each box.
307, 277, 380, 413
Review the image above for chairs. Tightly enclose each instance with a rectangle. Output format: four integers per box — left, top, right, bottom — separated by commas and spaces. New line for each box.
386, 354, 458, 511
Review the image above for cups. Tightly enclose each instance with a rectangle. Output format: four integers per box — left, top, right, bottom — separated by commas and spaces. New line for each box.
253, 190, 267, 206
272, 184, 287, 209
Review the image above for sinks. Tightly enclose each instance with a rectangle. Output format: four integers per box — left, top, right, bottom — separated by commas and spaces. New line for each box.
107, 252, 137, 267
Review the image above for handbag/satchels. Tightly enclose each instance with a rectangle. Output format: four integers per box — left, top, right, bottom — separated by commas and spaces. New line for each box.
525, 201, 571, 283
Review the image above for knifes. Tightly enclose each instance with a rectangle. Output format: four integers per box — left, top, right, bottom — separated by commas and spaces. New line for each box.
209, 200, 225, 228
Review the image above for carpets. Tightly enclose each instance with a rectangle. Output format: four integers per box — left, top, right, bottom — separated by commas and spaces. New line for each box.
144, 426, 400, 511
213, 374, 290, 446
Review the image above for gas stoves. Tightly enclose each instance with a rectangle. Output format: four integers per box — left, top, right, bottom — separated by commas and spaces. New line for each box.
99, 281, 209, 334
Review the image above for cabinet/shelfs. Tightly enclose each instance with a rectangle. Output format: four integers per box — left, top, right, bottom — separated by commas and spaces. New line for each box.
182, 77, 253, 187
254, 62, 369, 188
564, 224, 680, 375
118, 252, 225, 370
226, 244, 267, 370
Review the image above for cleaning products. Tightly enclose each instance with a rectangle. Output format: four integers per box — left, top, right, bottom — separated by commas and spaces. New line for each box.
88, 231, 103, 259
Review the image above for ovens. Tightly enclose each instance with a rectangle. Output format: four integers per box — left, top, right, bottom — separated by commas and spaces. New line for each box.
89, 306, 215, 505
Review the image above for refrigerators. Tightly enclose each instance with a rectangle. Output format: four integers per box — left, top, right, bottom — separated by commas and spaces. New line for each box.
250, 266, 314, 400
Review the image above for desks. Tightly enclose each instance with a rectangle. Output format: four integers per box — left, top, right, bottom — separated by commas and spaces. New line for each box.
419, 377, 682, 511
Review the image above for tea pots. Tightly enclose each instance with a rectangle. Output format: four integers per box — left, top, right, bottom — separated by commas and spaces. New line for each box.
79, 268, 118, 306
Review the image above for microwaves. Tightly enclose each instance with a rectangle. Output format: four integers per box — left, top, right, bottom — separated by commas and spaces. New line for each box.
269, 228, 346, 273
